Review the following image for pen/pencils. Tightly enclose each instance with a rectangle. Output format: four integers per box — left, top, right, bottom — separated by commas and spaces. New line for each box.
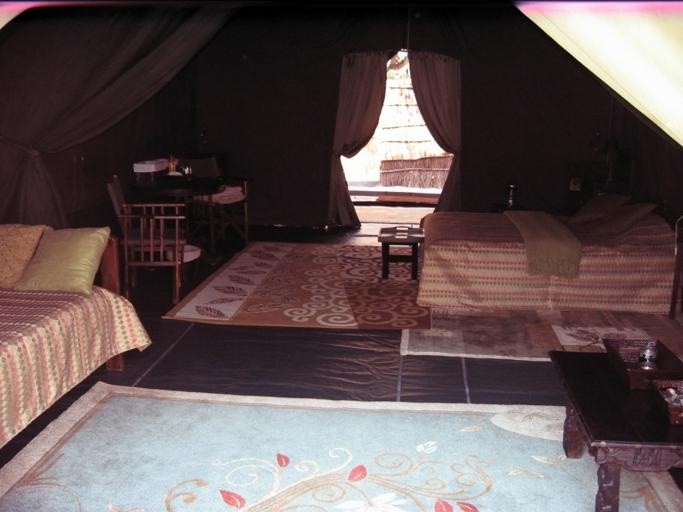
167, 152, 175, 171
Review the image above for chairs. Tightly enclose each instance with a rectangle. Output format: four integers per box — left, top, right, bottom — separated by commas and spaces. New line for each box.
107, 173, 187, 304
186, 153, 251, 247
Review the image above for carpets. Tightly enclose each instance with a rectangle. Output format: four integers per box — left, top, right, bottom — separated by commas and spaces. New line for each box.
0, 380, 683, 512
399, 315, 682, 362
161, 240, 432, 331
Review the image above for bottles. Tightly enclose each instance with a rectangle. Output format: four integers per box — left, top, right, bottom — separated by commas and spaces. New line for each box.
168, 154, 176, 174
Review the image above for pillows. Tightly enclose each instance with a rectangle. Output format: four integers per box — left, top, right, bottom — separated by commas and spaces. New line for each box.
570, 194, 629, 222
0, 224, 45, 289
587, 201, 657, 236
16, 227, 109, 294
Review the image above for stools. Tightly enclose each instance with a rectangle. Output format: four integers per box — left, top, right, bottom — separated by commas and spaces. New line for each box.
382, 243, 418, 279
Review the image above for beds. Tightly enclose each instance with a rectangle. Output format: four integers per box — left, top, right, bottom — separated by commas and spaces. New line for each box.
416, 210, 678, 314
0, 233, 124, 449
